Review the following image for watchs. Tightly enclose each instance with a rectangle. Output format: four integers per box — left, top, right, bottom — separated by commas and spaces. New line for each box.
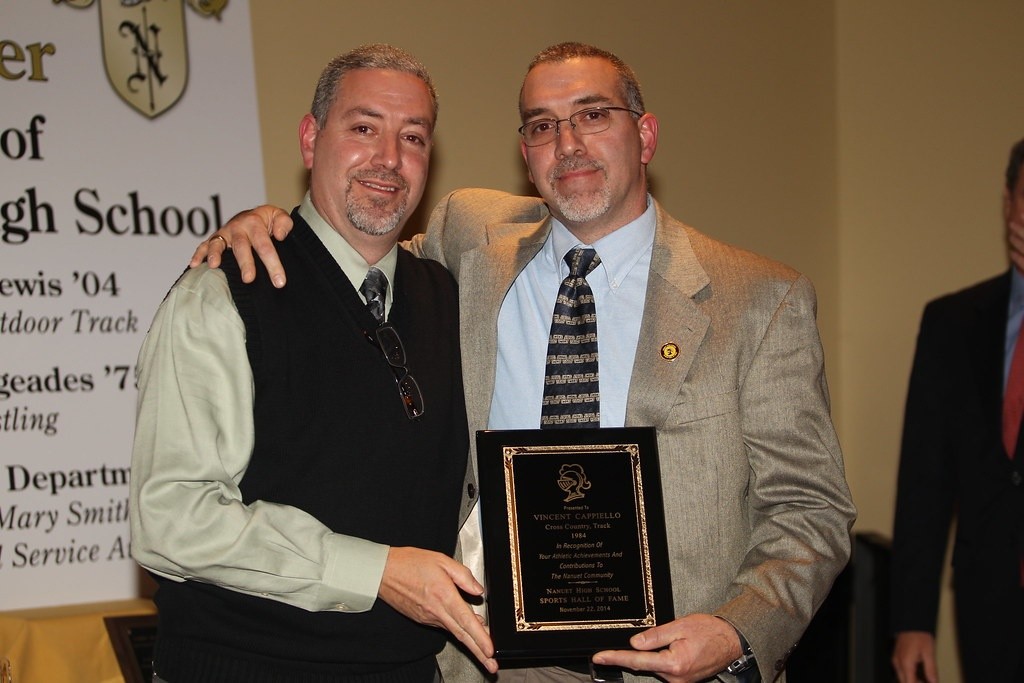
727, 627, 756, 675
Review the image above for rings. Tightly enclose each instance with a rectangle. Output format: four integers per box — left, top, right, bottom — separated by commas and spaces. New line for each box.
209, 235, 228, 250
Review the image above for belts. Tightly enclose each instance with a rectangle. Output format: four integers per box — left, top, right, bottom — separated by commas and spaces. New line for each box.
555, 658, 624, 683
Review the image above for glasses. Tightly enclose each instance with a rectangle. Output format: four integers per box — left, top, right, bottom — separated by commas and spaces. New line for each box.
376, 322, 425, 423
518, 105, 644, 147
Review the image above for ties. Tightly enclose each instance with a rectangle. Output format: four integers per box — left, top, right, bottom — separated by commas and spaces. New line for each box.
539, 247, 602, 431
358, 266, 389, 324
1002, 315, 1024, 464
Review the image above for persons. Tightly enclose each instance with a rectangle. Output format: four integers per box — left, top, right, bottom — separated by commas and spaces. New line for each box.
129, 43, 498, 683
883, 138, 1024, 683
192, 42, 858, 683
